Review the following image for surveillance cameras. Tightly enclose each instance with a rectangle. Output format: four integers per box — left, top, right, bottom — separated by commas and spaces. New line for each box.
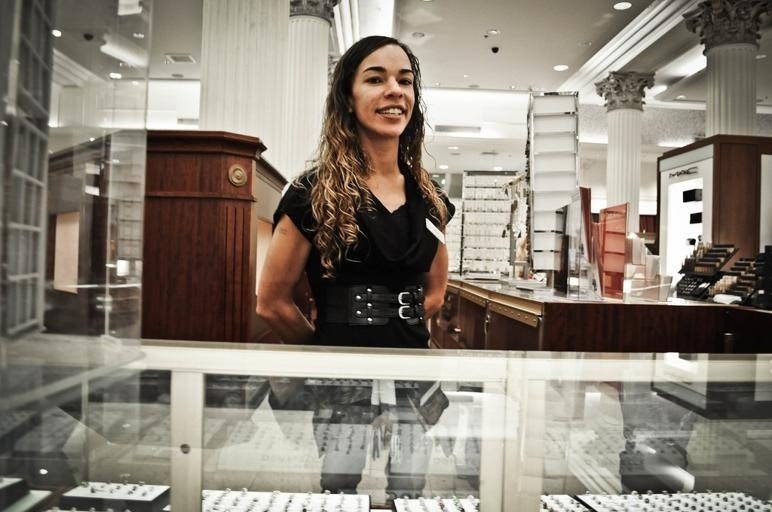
491, 47, 499, 53
84, 33, 93, 40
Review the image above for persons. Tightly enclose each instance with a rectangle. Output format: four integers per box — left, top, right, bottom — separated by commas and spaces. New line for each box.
253, 34, 456, 499
